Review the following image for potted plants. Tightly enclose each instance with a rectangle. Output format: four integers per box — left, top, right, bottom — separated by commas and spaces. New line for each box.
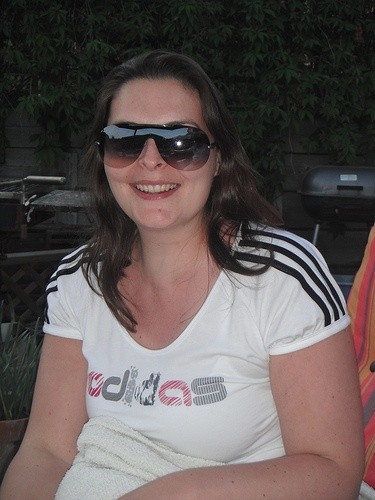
0, 320, 48, 479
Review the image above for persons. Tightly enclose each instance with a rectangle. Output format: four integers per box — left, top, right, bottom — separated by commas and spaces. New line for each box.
1, 49, 368, 499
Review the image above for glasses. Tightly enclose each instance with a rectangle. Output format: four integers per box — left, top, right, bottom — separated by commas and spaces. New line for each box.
95, 123, 214, 170
